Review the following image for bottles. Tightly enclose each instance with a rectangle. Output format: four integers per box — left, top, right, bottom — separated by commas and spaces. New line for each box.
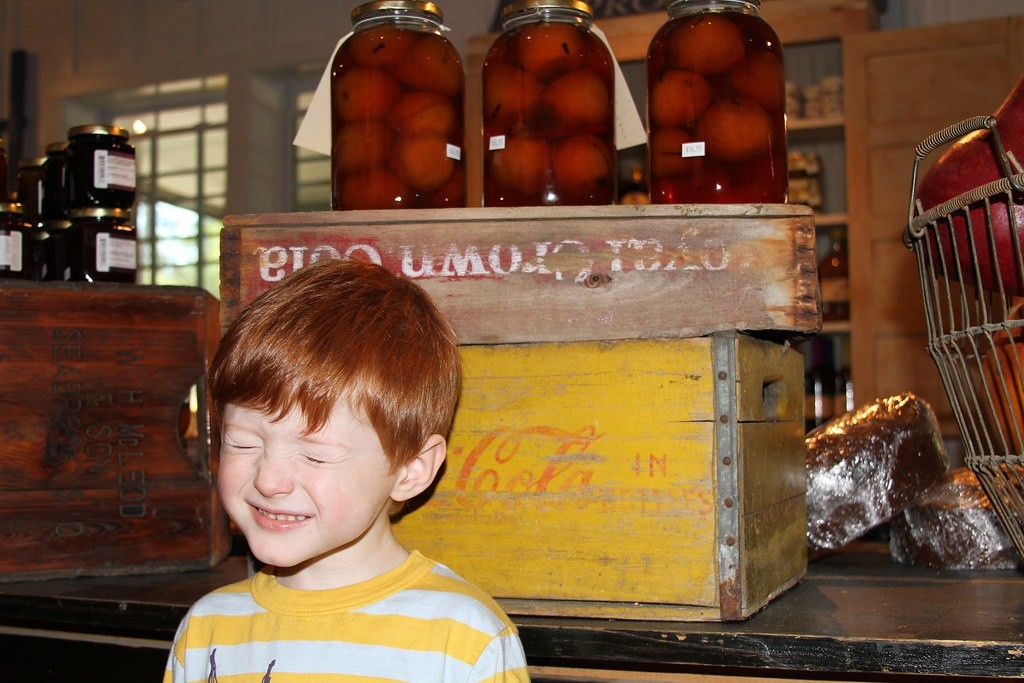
819, 229, 852, 320
833, 339, 851, 418
804, 338, 834, 433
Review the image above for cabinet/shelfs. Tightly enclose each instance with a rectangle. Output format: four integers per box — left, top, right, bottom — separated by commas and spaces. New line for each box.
466, 0, 884, 425
842, 16, 1024, 437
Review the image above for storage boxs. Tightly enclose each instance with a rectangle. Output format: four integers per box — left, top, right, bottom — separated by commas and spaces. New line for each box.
245, 336, 810, 623
0, 280, 238, 583
219, 205, 824, 345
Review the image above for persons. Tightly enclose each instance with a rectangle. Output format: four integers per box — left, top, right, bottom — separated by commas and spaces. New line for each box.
162, 258, 531, 683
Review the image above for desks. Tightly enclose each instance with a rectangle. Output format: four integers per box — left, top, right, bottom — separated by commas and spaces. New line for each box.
0, 540, 1024, 683
